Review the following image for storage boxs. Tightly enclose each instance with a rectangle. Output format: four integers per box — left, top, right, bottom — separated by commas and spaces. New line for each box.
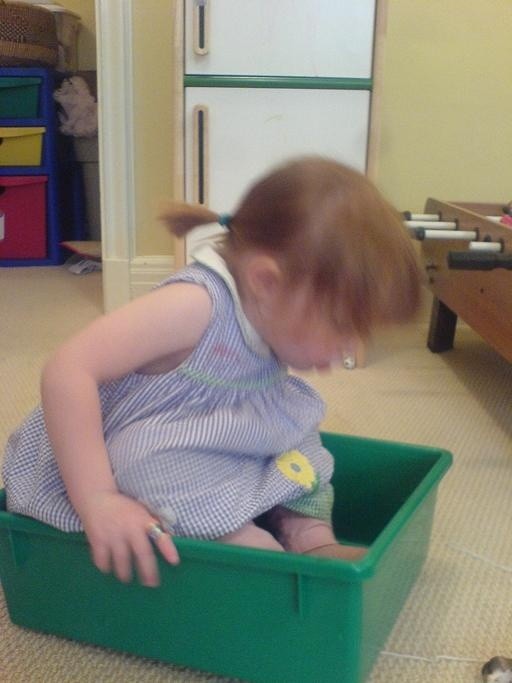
1, 432, 454, 682
0, 173, 51, 263
0, 67, 44, 119
0, 125, 49, 168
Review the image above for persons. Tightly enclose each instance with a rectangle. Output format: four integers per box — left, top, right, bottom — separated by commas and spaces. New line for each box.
1, 155, 427, 587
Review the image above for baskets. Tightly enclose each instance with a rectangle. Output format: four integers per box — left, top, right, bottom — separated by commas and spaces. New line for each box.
0, 0, 61, 67
